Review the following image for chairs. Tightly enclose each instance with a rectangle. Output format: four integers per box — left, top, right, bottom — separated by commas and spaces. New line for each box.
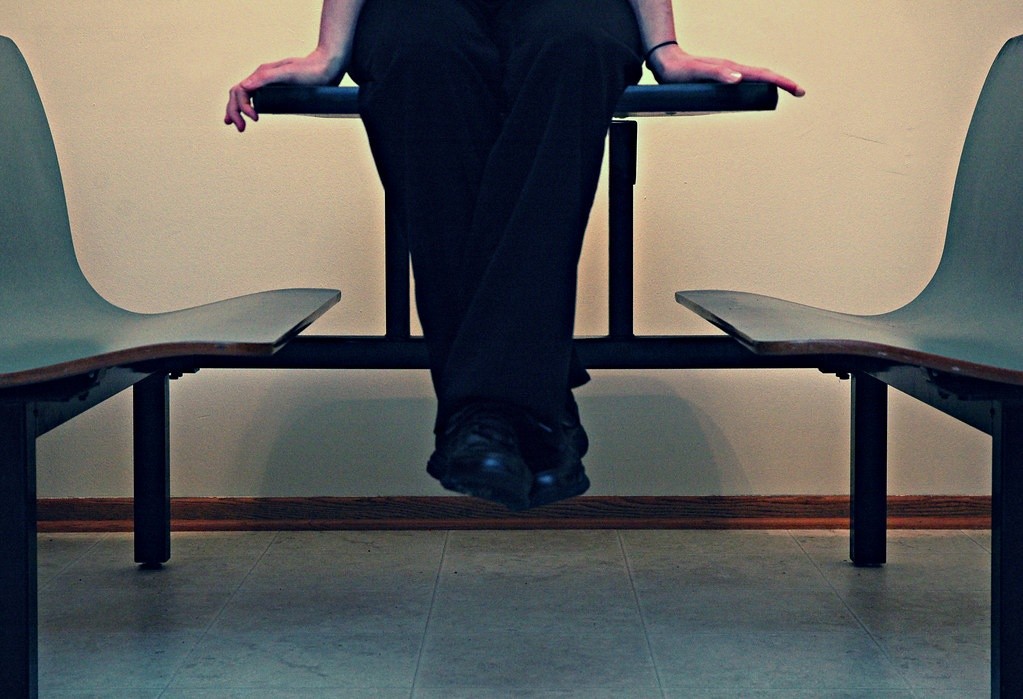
0, 35, 341, 699
676, 34, 1023, 699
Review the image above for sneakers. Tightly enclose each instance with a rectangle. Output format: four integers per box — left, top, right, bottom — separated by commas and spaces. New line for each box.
520, 423, 588, 507
427, 417, 531, 509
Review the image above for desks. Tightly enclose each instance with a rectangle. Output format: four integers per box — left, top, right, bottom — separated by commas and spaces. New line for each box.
253, 78, 780, 372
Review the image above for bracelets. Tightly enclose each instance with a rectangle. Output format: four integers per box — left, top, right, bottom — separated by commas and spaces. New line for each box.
646, 41, 677, 70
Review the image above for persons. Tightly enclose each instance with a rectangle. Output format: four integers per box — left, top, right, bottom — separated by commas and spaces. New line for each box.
224, 0, 806, 510
